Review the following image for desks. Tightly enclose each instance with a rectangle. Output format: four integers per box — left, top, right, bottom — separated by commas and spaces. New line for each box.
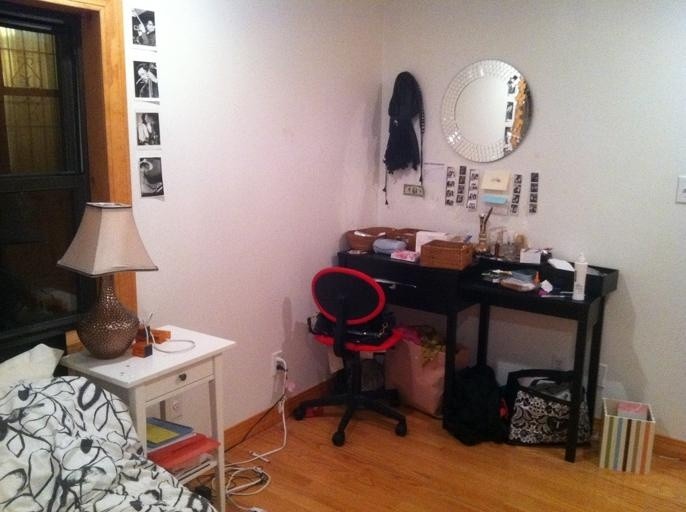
336, 240, 613, 465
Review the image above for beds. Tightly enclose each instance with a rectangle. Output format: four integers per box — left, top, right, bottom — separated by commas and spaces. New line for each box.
0, 341, 215, 512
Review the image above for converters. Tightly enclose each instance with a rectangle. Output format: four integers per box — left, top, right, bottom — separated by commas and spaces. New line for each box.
195, 485, 211, 500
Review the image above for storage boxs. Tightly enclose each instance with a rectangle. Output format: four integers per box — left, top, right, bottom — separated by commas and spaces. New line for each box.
597, 395, 659, 478
539, 259, 620, 298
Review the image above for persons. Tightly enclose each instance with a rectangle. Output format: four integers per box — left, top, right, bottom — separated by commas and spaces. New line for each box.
140, 162, 163, 195
136, 63, 157, 98
138, 113, 154, 145
137, 19, 154, 37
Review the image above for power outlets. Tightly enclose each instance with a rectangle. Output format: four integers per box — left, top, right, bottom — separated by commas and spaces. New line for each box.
271, 349, 286, 376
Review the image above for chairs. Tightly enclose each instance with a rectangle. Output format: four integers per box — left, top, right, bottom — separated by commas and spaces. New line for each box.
291, 266, 409, 446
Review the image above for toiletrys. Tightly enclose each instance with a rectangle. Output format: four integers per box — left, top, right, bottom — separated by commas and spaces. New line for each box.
571, 253, 588, 301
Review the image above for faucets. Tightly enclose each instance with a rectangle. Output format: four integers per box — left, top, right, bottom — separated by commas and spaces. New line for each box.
477, 206, 492, 244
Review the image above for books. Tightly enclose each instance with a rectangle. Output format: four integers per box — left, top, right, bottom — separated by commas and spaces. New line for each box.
145, 417, 199, 453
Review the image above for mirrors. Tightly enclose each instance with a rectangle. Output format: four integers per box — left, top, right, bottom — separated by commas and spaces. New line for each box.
438, 58, 536, 163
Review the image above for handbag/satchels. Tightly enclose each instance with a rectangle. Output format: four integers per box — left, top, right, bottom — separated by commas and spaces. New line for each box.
307, 310, 399, 345
505, 367, 593, 447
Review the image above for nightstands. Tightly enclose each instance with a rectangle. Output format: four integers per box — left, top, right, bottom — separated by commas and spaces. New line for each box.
58, 326, 238, 511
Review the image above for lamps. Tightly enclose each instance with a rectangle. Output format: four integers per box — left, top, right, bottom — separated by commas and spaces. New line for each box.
54, 199, 162, 358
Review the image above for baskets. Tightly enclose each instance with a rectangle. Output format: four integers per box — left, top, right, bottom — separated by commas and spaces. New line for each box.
347, 226, 432, 252
